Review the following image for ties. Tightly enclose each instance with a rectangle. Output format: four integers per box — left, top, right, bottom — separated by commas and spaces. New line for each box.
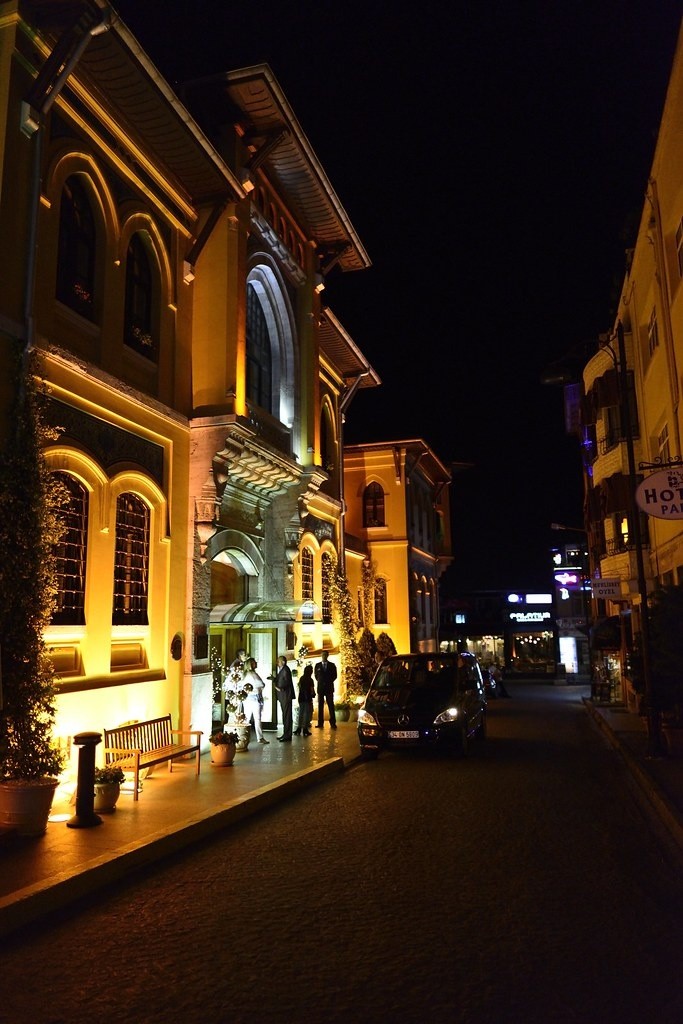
324, 662, 325, 671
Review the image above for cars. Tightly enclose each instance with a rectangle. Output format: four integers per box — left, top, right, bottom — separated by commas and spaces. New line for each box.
480, 669, 497, 698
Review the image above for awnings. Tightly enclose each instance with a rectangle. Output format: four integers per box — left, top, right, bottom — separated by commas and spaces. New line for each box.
589, 614, 621, 651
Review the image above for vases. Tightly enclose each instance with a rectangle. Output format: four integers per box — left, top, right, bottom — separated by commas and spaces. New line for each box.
210, 744, 236, 762
94, 783, 120, 809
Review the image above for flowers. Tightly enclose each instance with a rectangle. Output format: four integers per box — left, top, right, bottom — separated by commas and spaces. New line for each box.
209, 731, 240, 746
93, 765, 126, 784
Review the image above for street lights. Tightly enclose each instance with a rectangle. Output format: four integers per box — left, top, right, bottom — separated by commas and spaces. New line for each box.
542, 339, 662, 758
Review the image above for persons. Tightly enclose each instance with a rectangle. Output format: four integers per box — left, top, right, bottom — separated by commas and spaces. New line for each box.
266, 656, 296, 742
314, 650, 337, 729
226, 648, 270, 744
293, 664, 317, 736
371, 650, 385, 672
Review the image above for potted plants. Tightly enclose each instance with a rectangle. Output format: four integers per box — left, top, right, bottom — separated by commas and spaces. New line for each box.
0, 323, 75, 832
335, 701, 361, 722
222, 656, 255, 747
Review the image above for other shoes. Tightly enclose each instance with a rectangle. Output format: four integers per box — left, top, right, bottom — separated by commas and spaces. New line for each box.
293, 730, 301, 735
258, 738, 269, 744
280, 737, 289, 741
304, 731, 312, 735
331, 723, 337, 728
315, 724, 323, 727
277, 734, 285, 739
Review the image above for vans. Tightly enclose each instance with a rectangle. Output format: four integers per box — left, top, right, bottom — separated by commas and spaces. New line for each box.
356, 651, 485, 761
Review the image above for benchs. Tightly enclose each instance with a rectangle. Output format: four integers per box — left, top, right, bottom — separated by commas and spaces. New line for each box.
103, 713, 204, 800
